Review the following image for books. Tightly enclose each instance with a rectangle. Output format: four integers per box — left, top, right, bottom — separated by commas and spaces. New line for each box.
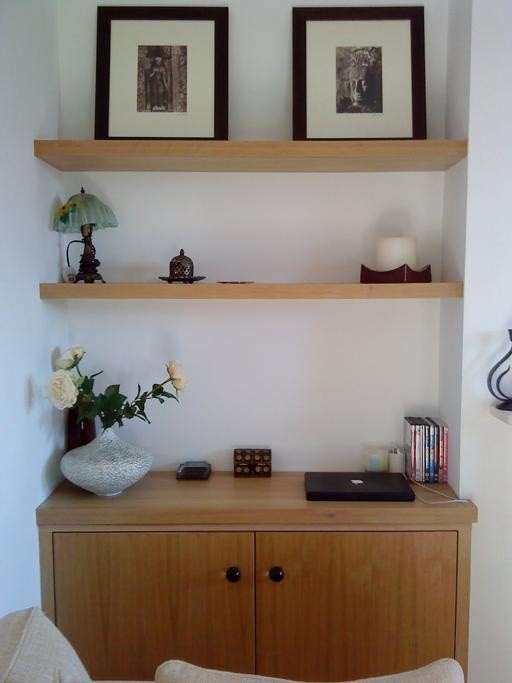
402, 415, 448, 484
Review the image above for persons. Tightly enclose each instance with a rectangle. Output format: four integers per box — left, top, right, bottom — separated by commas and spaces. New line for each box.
340, 65, 379, 112
149, 55, 169, 110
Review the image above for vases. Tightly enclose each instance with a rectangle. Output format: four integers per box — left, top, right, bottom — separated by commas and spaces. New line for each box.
58, 428, 153, 497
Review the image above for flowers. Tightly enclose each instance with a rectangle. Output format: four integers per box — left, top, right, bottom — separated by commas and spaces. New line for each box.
46, 340, 187, 429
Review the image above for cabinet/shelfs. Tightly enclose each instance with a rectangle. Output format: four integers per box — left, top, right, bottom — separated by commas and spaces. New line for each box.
36, 469, 476, 681
33, 141, 470, 297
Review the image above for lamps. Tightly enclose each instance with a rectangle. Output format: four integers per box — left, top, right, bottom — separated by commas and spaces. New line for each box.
52, 187, 118, 284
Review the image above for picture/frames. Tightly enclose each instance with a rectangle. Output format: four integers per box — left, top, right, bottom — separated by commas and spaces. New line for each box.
292, 7, 427, 140
95, 5, 229, 139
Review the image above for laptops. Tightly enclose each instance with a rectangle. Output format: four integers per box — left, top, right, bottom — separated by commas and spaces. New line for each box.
304, 471, 416, 502
